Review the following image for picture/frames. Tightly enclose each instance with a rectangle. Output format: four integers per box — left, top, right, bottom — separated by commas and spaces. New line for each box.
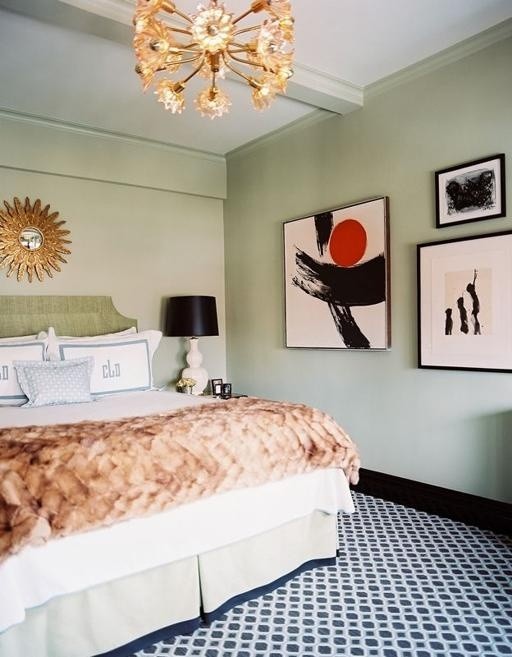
215, 384, 222, 395
415, 230, 511, 371
212, 378, 222, 394
433, 152, 507, 228
282, 194, 391, 351
222, 382, 232, 395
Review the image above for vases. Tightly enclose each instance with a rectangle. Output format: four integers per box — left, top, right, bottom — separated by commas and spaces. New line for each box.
180, 385, 192, 395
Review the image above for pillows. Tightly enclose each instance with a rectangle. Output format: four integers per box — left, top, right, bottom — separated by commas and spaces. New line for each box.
1, 330, 48, 343
12, 354, 97, 408
0, 335, 51, 406
50, 325, 137, 341
48, 325, 163, 398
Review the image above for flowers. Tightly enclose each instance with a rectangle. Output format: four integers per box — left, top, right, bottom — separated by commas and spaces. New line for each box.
175, 376, 199, 387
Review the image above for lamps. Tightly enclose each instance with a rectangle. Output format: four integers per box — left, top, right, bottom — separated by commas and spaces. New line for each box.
162, 295, 220, 393
1, 194, 72, 284
132, 0, 297, 123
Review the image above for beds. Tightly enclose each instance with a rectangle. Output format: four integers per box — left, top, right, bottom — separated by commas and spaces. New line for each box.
0, 296, 363, 657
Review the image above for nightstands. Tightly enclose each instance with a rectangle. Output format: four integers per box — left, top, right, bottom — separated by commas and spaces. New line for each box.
202, 393, 249, 398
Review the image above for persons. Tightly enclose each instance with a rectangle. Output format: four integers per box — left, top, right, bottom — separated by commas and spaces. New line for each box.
442, 273, 482, 334
